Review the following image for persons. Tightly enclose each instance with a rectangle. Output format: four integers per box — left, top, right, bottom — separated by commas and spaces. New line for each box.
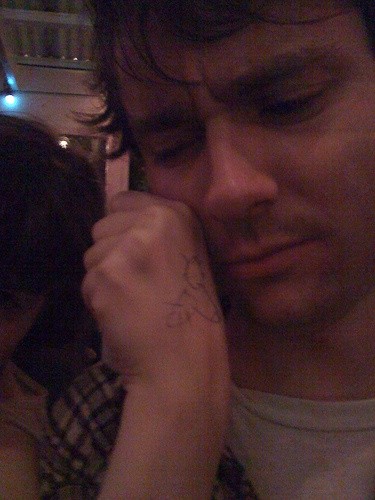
0, 112, 231, 500
35, 0, 375, 500
